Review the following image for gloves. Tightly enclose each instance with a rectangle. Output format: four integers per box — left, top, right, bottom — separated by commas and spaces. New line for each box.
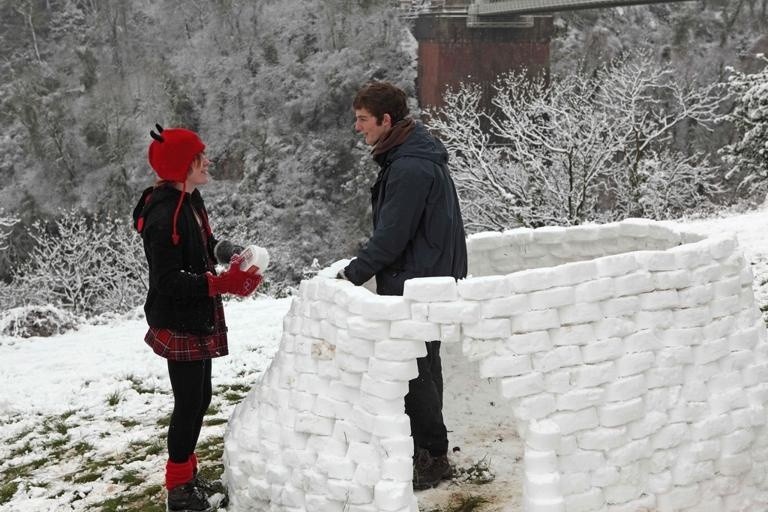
214, 239, 245, 263
205, 258, 262, 296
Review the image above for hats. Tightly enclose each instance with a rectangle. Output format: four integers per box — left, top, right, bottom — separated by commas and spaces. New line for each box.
149, 124, 205, 183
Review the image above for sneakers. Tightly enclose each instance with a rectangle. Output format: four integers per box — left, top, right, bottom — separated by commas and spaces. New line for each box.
413, 447, 452, 490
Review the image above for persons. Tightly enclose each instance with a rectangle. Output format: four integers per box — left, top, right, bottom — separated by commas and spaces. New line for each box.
335, 83, 469, 494
133, 124, 263, 512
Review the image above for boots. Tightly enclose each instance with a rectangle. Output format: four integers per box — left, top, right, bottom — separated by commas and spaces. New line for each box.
168, 468, 229, 512
